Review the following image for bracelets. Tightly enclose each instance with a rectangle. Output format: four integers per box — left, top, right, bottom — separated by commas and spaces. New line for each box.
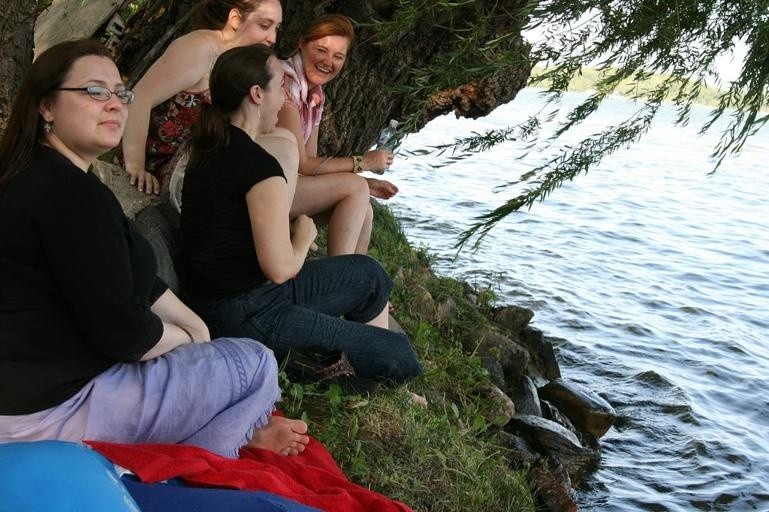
350, 154, 364, 175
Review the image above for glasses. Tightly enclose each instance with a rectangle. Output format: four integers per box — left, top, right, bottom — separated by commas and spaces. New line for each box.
57, 86, 136, 105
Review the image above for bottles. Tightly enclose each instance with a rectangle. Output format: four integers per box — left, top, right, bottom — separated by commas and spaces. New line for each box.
371, 120, 398, 174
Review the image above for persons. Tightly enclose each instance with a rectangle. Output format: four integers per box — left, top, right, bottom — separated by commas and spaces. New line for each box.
181, 44, 429, 406
273, 13, 402, 258
109, 0, 288, 197
0, 39, 308, 461
